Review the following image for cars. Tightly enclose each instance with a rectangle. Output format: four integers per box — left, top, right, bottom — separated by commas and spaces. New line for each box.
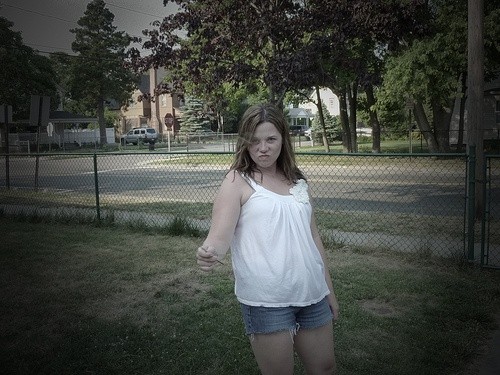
289, 125, 308, 136
304, 127, 312, 140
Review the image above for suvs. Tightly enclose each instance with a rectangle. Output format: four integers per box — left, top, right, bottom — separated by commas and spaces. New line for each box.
120, 127, 158, 145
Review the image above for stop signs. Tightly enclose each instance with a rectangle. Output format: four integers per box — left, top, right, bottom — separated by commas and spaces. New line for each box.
164, 113, 175, 129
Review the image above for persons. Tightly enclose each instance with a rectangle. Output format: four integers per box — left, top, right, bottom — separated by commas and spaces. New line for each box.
195, 107, 341, 375
